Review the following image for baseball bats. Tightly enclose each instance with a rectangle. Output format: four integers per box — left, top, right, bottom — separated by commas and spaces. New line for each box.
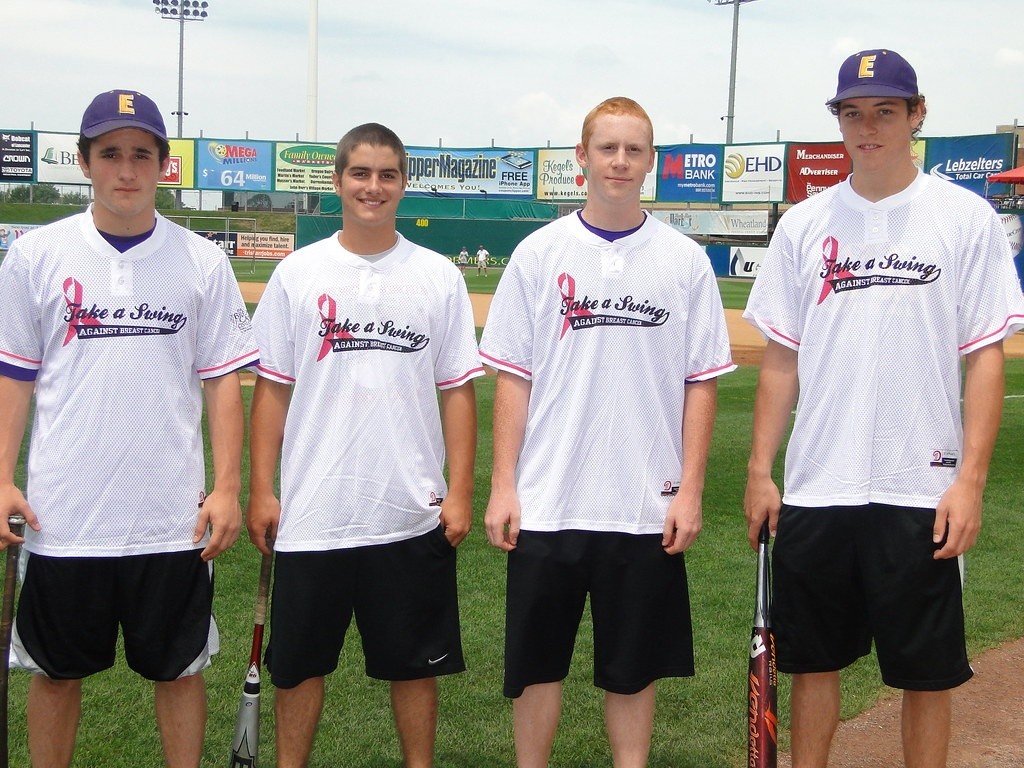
746, 517, 780, 768
0, 515, 27, 768
228, 537, 278, 768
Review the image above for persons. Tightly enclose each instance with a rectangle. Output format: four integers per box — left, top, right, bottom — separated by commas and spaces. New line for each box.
476, 245, 489, 277
244, 123, 486, 768
479, 97, 738, 768
459, 246, 468, 277
0, 89, 261, 768
742, 50, 1024, 768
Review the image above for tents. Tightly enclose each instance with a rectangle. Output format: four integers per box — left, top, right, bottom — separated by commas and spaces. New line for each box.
985, 166, 1024, 199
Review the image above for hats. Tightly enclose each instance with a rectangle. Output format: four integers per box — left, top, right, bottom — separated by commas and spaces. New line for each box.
825, 49, 918, 105
79, 90, 169, 143
